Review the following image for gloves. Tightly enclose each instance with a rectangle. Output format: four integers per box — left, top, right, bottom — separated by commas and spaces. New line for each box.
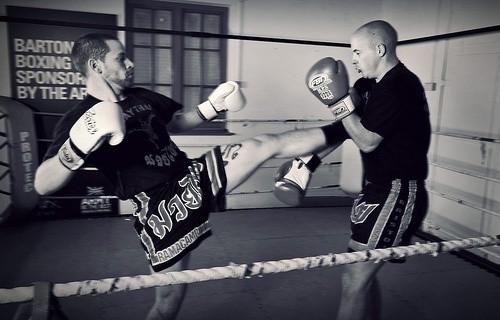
273, 152, 323, 207
197, 81, 246, 122
306, 57, 356, 122
58, 101, 127, 172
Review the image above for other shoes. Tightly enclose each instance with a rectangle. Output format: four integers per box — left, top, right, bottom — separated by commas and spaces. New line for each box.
353, 77, 376, 132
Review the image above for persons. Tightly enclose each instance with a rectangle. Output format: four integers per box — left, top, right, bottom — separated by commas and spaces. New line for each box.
274, 20, 431, 320
34, 32, 372, 320
